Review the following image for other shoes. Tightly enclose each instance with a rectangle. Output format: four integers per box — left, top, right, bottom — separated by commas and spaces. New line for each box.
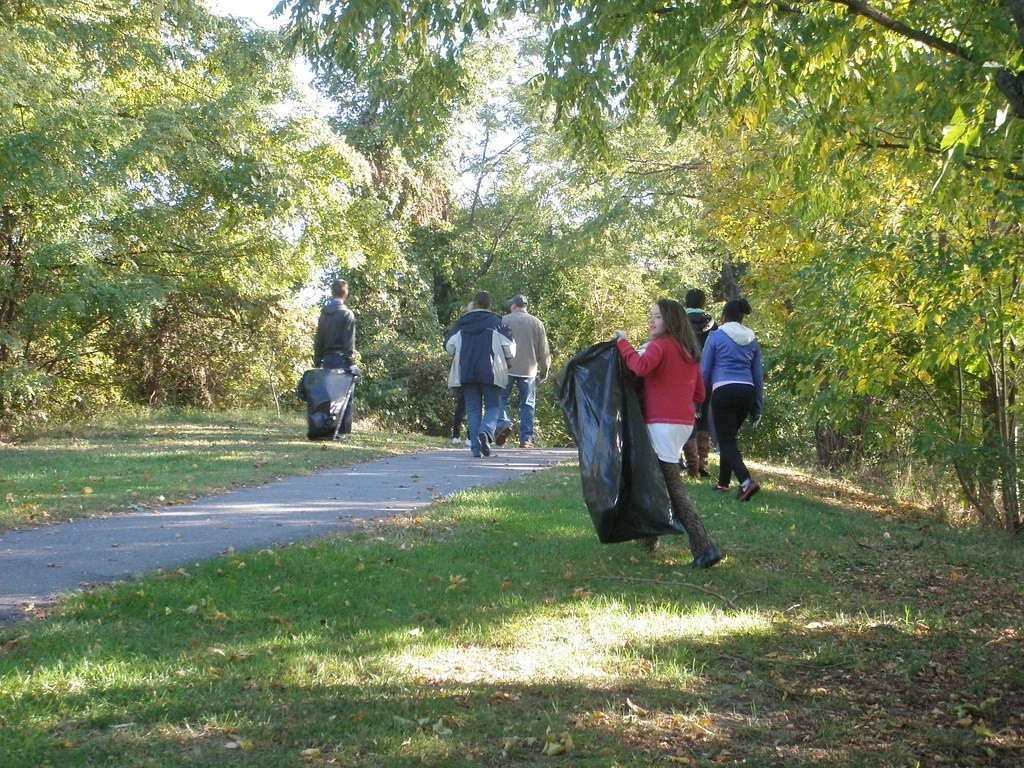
636, 536, 657, 552
452, 437, 463, 444
689, 545, 721, 569
465, 438, 472, 446
699, 467, 710, 477
519, 442, 533, 449
478, 431, 491, 456
495, 425, 512, 446
473, 451, 482, 457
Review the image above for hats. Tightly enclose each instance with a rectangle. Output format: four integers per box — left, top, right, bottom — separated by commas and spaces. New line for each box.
508, 295, 529, 307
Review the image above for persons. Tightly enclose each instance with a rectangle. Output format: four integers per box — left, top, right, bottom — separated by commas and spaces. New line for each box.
313, 279, 362, 439
701, 295, 764, 502
492, 294, 552, 449
442, 292, 517, 458
451, 387, 472, 446
676, 287, 720, 479
611, 298, 723, 570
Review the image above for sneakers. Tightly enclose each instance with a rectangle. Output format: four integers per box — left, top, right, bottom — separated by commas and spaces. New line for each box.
715, 482, 729, 492
737, 479, 760, 501
